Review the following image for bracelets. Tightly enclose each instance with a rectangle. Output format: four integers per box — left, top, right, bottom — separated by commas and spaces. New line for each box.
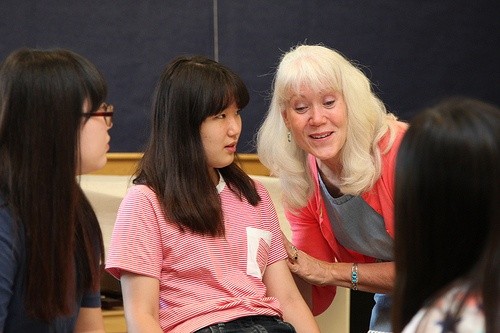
351, 262, 359, 291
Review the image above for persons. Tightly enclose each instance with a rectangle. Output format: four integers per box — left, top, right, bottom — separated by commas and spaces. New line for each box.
256, 45, 411, 333
391, 97, 500, 333
0, 48, 114, 333
105, 56, 319, 333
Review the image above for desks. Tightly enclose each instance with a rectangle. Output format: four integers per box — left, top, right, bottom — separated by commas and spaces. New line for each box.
77, 153, 272, 175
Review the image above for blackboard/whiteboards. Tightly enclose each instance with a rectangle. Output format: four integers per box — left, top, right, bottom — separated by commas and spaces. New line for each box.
0, 0, 500, 161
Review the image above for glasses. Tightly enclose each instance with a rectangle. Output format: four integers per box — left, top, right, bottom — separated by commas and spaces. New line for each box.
81, 100, 115, 127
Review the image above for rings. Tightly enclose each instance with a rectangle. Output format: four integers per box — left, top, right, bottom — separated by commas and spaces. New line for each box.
291, 246, 297, 251
291, 252, 298, 261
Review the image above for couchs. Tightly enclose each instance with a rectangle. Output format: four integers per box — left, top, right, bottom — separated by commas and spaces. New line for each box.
72, 176, 349, 333
